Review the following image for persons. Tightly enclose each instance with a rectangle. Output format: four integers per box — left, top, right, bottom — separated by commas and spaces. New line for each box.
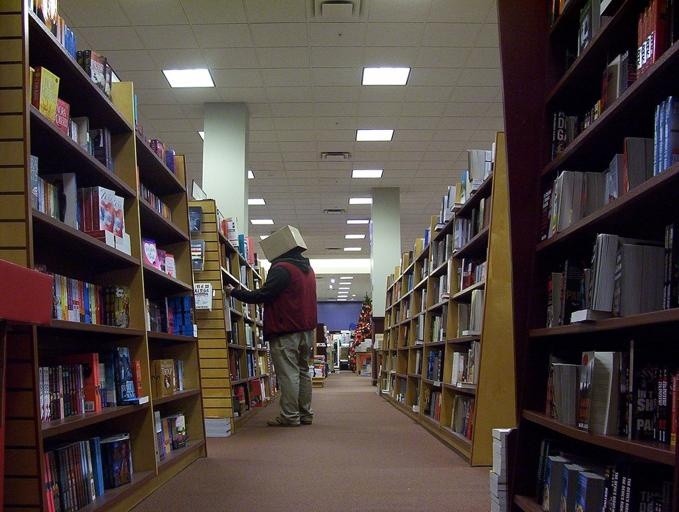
221, 225, 316, 427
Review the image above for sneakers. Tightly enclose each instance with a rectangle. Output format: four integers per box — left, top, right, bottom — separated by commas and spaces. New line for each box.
266, 411, 313, 427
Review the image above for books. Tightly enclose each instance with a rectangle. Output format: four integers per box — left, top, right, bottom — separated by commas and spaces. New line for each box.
150, 359, 183, 399
29, 156, 131, 255
379, 144, 494, 444
141, 239, 197, 337
218, 212, 271, 417
532, 0, 677, 511
36, 267, 130, 327
154, 411, 187, 461
133, 96, 175, 222
194, 283, 213, 312
313, 352, 326, 378
189, 206, 205, 272
39, 347, 150, 422
27, 0, 113, 171
44, 430, 133, 511
488, 428, 510, 511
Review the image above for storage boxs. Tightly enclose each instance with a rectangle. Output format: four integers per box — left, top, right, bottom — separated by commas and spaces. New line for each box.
258, 223, 310, 263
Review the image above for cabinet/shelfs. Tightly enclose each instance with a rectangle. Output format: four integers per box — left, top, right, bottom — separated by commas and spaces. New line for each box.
312, 319, 373, 391
376, 0, 678, 512
0, 1, 281, 512
